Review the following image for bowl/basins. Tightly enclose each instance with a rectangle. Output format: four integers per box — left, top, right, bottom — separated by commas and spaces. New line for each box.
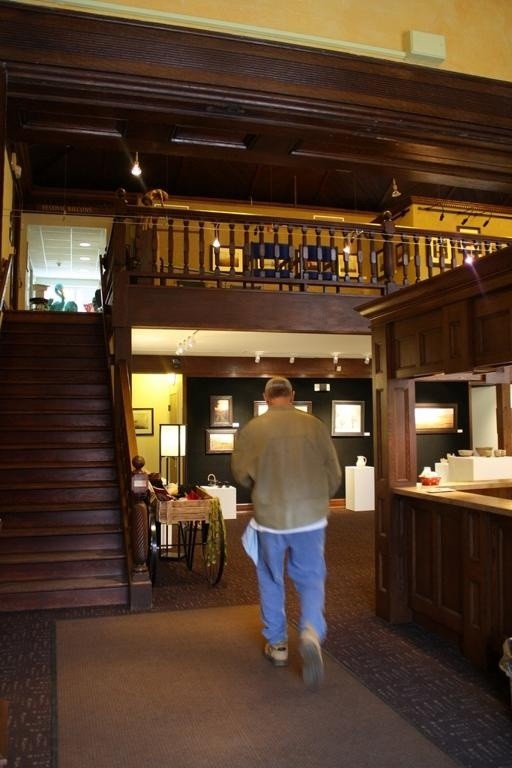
417, 476, 441, 486
475, 447, 493, 456
457, 449, 473, 457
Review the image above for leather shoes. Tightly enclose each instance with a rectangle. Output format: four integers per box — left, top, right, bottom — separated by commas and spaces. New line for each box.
298, 629, 325, 689
264, 642, 288, 667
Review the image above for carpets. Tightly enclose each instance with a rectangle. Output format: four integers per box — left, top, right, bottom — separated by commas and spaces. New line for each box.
51, 605, 460, 767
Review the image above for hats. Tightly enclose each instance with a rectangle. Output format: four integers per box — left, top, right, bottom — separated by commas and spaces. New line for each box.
265, 376, 293, 392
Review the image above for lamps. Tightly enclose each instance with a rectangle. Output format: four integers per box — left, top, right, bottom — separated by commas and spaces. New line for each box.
343, 239, 352, 254
130, 152, 142, 175
157, 424, 189, 560
391, 178, 401, 197
212, 224, 220, 247
254, 351, 372, 365
417, 205, 494, 227
174, 330, 200, 355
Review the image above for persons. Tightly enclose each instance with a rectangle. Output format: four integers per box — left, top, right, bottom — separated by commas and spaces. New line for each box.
227, 375, 343, 695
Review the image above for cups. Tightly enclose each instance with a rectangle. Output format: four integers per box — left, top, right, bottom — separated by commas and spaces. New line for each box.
208, 480, 231, 488
494, 450, 506, 457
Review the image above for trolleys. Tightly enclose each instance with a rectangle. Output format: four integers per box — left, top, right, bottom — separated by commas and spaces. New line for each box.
146, 479, 230, 590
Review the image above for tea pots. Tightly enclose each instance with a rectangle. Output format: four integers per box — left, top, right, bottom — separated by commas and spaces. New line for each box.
356, 455, 368, 467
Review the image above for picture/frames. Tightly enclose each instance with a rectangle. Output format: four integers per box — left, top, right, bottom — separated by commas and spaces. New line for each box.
210, 395, 233, 428
425, 224, 483, 268
414, 402, 458, 434
205, 429, 238, 454
376, 242, 410, 280
132, 408, 154, 436
331, 399, 365, 437
210, 244, 359, 286
253, 401, 312, 418
